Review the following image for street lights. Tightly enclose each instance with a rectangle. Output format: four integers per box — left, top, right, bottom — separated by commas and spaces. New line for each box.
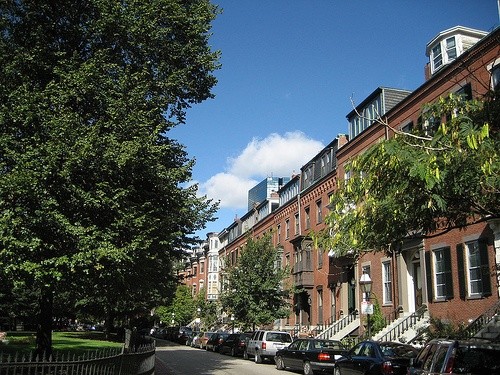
359, 267, 374, 342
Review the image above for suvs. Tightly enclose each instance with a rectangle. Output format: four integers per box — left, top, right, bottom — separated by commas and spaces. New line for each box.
332, 341, 419, 375
406, 334, 500, 375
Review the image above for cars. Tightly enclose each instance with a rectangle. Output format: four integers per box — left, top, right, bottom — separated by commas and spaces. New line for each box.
274, 338, 348, 375
149, 325, 253, 357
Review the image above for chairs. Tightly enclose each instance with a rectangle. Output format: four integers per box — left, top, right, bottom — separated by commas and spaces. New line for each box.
276, 335, 281, 340
329, 344, 334, 348
333, 346, 340, 349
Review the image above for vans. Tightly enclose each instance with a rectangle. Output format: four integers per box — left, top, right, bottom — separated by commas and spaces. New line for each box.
244, 330, 295, 363
